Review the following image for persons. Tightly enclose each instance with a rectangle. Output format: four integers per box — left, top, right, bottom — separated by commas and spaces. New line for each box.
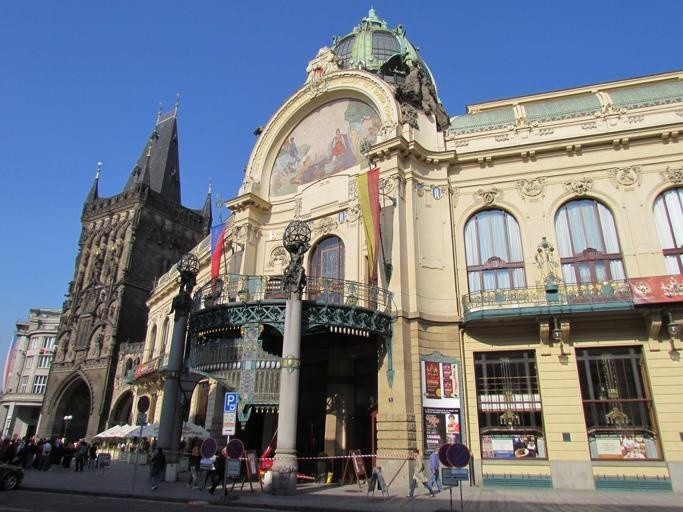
0, 434, 229, 498
445, 414, 459, 433
426, 447, 442, 493
406, 449, 434, 498
282, 114, 372, 165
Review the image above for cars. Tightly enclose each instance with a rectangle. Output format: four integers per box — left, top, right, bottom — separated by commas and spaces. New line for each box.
0, 459, 25, 491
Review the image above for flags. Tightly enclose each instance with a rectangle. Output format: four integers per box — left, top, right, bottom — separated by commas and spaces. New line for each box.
209, 221, 227, 290
354, 166, 380, 283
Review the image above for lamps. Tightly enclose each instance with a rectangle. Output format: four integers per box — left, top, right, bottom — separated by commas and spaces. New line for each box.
533, 234, 562, 299
548, 317, 564, 341
663, 309, 678, 337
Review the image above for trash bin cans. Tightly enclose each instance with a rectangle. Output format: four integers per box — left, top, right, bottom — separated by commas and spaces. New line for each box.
165, 462, 177, 483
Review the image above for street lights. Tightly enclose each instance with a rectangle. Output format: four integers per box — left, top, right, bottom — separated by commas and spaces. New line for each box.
62, 415, 74, 438
